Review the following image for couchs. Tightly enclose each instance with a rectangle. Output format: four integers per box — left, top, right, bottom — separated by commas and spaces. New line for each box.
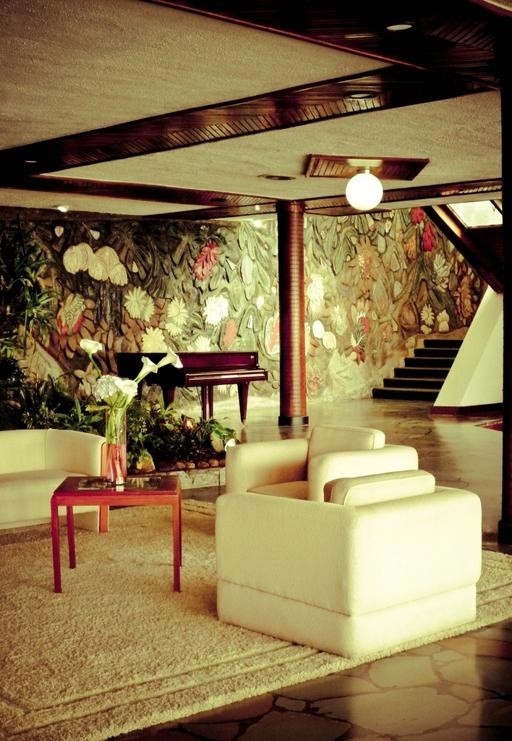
0, 427, 110, 534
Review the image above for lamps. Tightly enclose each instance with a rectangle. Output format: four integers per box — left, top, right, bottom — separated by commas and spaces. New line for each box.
345, 166, 384, 212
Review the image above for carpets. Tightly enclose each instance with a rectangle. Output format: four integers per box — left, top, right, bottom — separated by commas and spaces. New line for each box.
0, 497, 512, 741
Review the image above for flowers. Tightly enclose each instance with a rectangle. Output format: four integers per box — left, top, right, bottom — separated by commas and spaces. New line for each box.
79, 335, 184, 481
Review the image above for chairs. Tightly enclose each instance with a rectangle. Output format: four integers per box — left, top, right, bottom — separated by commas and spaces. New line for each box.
214, 469, 483, 660
224, 424, 419, 502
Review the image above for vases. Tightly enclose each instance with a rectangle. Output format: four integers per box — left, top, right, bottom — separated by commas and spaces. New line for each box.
105, 407, 127, 485
